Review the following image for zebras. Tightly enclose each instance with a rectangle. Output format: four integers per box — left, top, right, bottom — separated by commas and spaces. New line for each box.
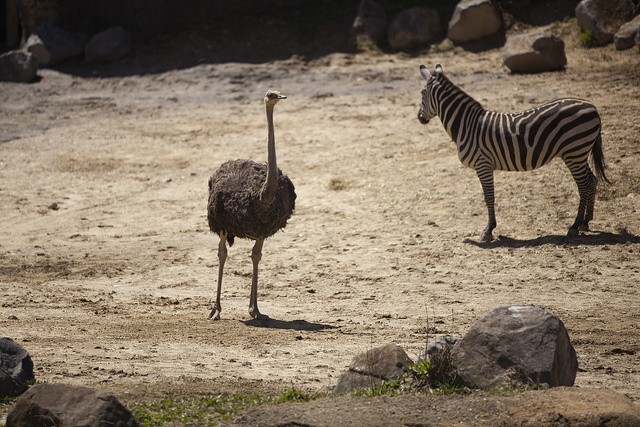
417, 62, 615, 243
